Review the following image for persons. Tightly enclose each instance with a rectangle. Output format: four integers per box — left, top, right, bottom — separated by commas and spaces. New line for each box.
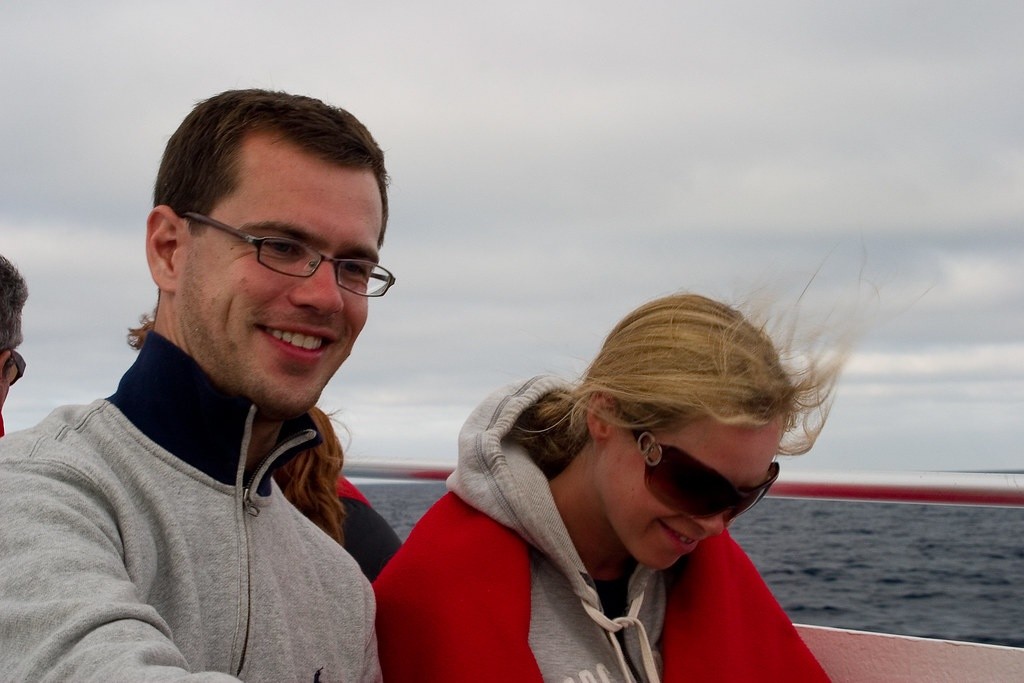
0, 255, 28, 438
372, 293, 833, 683
129, 320, 403, 582
0, 89, 388, 683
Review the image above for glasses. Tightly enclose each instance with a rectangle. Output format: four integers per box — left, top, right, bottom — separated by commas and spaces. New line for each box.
181, 212, 395, 298
5, 349, 26, 386
624, 414, 781, 525
